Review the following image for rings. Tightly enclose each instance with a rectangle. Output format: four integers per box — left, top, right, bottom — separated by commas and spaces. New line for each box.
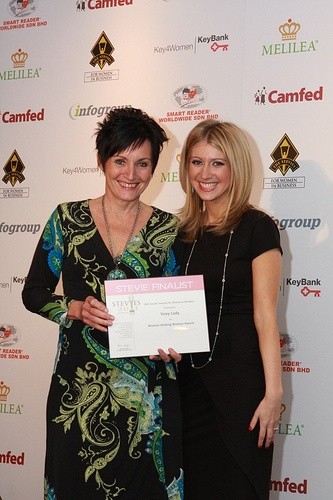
273, 428, 278, 431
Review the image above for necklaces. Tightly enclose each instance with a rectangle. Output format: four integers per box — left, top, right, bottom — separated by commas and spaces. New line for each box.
184, 229, 233, 369
102, 194, 141, 280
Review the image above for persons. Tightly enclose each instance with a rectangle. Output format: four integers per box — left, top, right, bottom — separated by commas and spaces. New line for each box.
173, 119, 283, 500
21, 107, 184, 500
